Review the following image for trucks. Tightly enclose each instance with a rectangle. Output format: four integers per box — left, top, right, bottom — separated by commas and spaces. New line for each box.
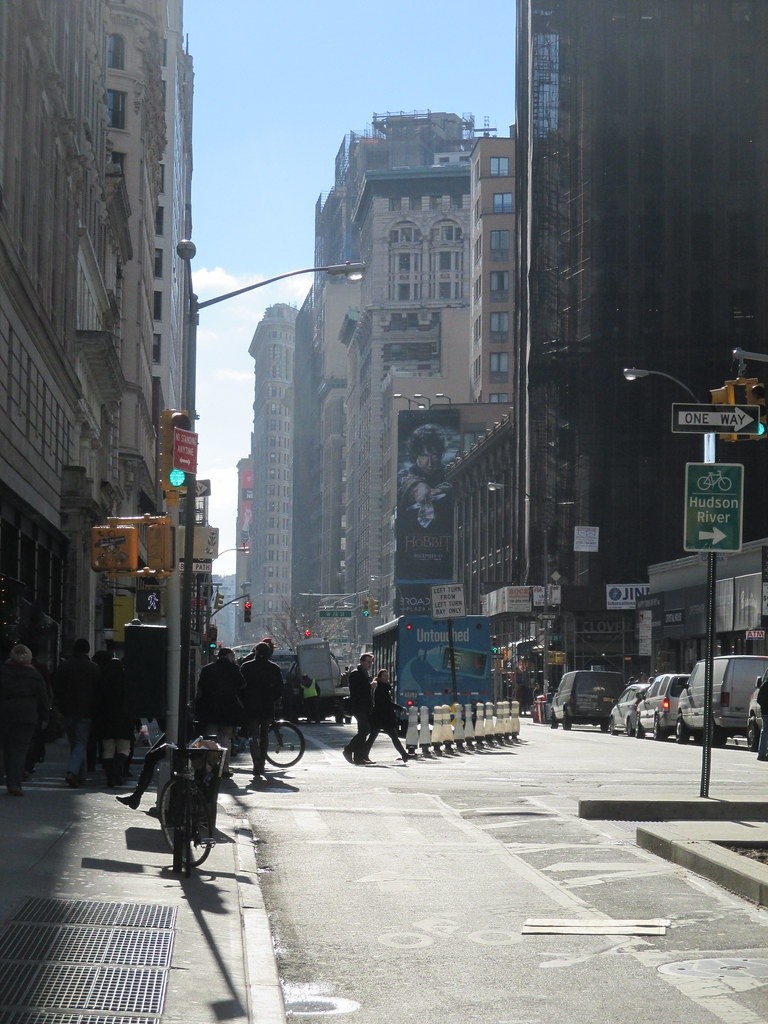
273, 639, 353, 724
372, 612, 490, 733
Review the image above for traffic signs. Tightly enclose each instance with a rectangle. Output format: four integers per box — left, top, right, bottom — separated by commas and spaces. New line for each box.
163, 480, 213, 499
684, 462, 744, 553
671, 402, 760, 434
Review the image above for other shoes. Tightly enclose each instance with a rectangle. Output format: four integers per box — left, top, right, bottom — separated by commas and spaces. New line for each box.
6, 786, 25, 797
343, 749, 371, 764
223, 771, 233, 778
362, 754, 377, 764
402, 753, 415, 763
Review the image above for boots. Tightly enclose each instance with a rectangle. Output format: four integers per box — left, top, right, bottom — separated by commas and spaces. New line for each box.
116, 766, 155, 810
102, 753, 128, 787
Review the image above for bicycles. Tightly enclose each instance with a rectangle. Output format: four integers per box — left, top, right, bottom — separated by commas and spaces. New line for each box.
150, 741, 219, 876
201, 714, 306, 768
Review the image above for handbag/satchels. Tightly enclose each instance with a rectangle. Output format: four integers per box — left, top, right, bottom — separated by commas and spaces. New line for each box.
342, 697, 352, 718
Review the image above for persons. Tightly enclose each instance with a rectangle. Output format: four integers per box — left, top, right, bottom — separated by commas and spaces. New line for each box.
508, 680, 553, 717
0, 639, 415, 810
398, 424, 454, 579
628, 672, 655, 684
757, 679, 768, 761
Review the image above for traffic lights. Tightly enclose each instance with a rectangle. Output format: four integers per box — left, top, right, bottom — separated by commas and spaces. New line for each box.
160, 411, 193, 493
90, 519, 171, 573
207, 624, 217, 654
244, 601, 251, 622
214, 591, 224, 609
363, 599, 380, 618
136, 587, 163, 615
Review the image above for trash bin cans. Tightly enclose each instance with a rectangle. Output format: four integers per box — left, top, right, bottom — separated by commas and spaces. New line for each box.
157, 749, 228, 827
533, 693, 553, 724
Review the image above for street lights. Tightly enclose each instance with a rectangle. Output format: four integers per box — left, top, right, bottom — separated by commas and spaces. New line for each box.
488, 482, 553, 722
211, 547, 251, 562
150, 260, 371, 803
623, 364, 719, 789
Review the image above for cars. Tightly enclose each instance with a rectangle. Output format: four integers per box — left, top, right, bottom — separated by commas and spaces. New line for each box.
748, 666, 768, 761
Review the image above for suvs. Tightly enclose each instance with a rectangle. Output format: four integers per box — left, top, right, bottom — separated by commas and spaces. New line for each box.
550, 669, 622, 732
607, 672, 691, 740
675, 653, 768, 748
